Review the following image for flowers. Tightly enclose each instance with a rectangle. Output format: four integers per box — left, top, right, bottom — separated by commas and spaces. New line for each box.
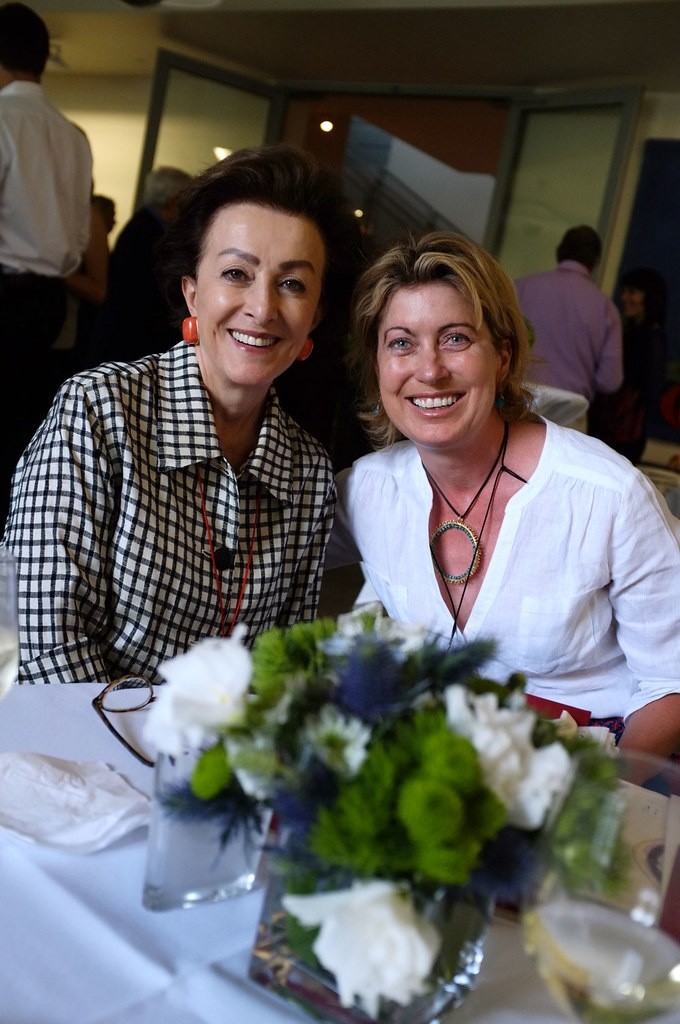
148, 614, 626, 1014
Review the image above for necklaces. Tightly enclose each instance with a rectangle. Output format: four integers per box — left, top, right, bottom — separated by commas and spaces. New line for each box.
421, 416, 509, 585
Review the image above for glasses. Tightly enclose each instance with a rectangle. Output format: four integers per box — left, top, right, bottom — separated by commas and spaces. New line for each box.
92, 674, 157, 768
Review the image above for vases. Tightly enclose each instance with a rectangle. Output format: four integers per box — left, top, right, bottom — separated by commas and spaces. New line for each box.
248, 855, 489, 1023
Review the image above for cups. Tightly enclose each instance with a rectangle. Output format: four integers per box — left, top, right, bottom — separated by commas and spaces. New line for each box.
520, 750, 680, 1024
141, 627, 268, 913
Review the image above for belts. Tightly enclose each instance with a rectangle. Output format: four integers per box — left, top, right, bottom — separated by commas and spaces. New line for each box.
0, 273, 59, 285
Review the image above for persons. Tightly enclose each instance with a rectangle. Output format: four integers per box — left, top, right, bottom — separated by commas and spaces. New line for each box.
0, 0, 116, 493
4, 140, 344, 695
584, 273, 667, 467
100, 168, 198, 357
513, 227, 623, 435
322, 232, 680, 796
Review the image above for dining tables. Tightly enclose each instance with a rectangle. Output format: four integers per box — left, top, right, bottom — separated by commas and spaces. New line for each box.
1, 686, 680, 1023
